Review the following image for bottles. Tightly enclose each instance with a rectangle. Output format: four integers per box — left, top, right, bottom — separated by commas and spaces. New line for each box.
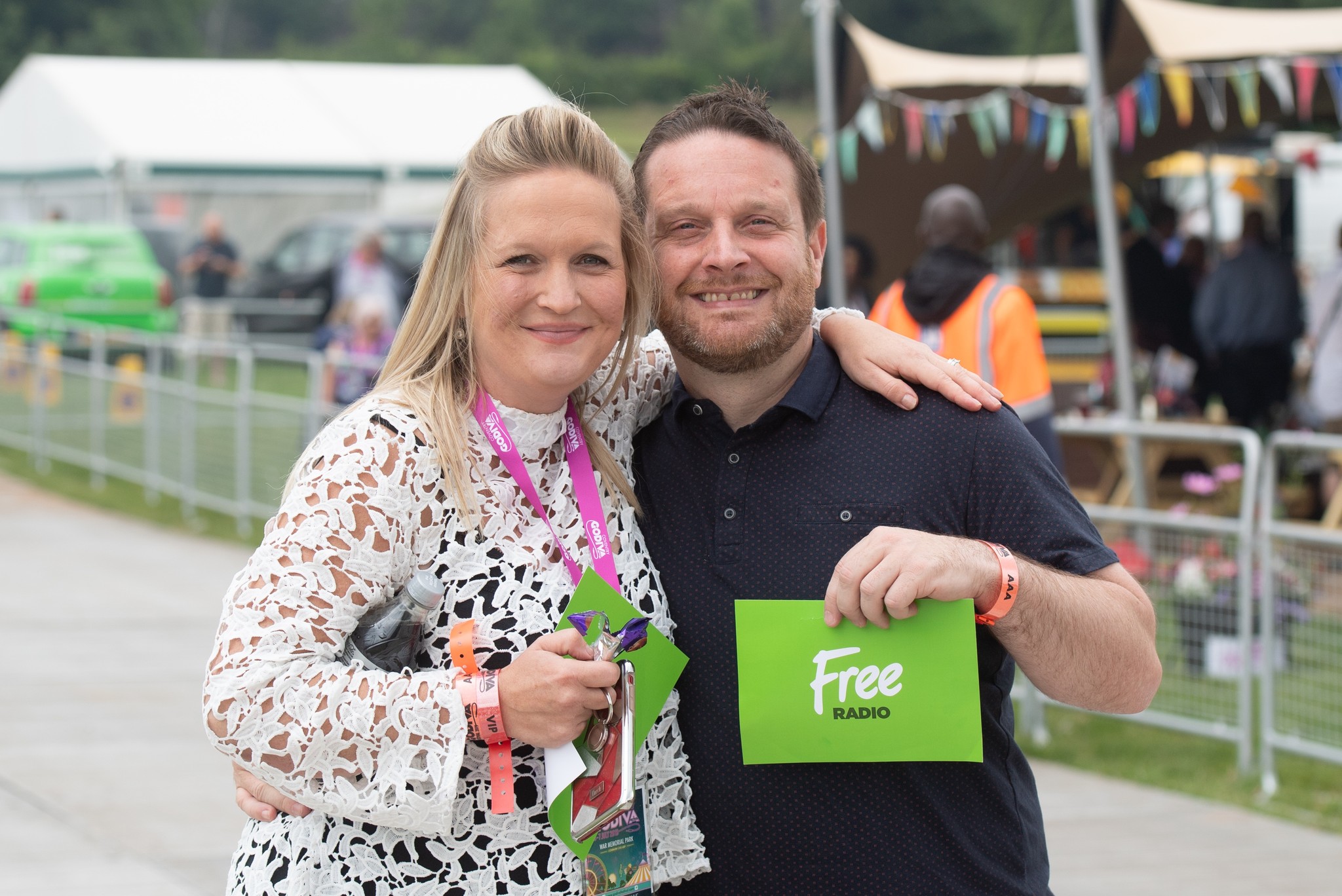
336, 571, 446, 675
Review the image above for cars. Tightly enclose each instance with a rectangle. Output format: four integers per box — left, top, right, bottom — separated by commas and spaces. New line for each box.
0, 219, 179, 373
232, 212, 440, 344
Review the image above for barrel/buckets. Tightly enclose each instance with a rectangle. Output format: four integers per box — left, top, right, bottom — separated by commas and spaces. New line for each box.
1181, 595, 1294, 675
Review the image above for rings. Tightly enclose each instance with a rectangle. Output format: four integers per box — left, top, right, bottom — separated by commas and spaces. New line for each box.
946, 357, 960, 368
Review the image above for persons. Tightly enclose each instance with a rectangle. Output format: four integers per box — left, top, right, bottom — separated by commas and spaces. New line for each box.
817, 230, 880, 319
178, 212, 240, 390
1029, 199, 1308, 495
199, 99, 1008, 895
314, 233, 399, 408
234, 90, 1163, 895
864, 185, 1062, 484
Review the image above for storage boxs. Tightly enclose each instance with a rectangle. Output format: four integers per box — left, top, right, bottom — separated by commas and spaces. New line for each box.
1176, 599, 1296, 680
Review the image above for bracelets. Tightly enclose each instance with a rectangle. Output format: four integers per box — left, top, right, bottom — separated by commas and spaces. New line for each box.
971, 537, 1019, 628
450, 618, 483, 743
476, 667, 519, 814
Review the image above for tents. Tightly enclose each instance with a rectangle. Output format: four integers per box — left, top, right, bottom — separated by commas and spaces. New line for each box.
0, 50, 576, 268
749, 1, 1342, 159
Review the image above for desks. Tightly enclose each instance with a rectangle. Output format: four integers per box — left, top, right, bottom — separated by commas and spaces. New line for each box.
1089, 420, 1248, 555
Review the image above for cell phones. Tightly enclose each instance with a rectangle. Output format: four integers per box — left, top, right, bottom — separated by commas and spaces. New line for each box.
570, 659, 636, 843
